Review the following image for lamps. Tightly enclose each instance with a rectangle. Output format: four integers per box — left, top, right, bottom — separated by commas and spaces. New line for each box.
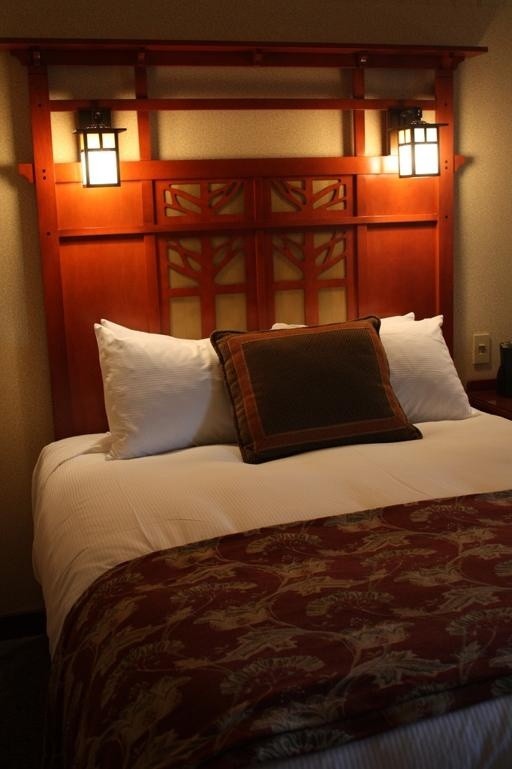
71, 101, 128, 188
380, 105, 449, 180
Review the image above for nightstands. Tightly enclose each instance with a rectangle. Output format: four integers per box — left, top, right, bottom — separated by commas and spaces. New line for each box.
464, 378, 512, 420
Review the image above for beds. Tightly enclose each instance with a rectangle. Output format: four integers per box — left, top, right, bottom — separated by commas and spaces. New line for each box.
33, 405, 512, 767
